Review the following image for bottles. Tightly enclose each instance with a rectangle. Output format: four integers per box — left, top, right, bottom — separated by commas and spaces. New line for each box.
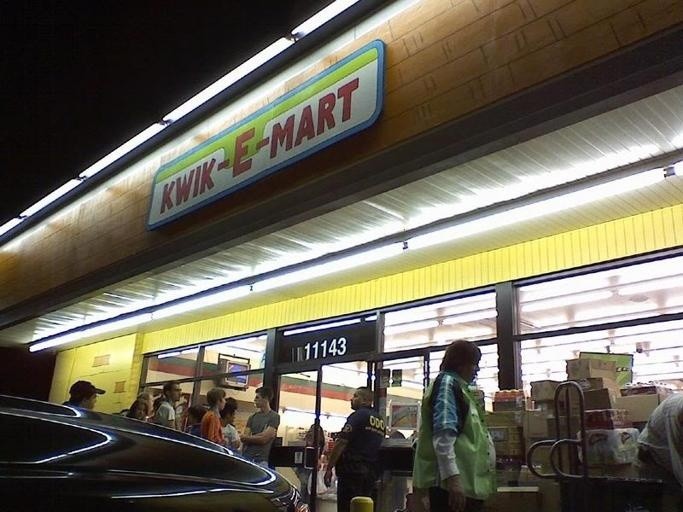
519, 464, 543, 486
493, 389, 524, 402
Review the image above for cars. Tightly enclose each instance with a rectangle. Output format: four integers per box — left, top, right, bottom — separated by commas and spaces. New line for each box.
0, 391, 311, 512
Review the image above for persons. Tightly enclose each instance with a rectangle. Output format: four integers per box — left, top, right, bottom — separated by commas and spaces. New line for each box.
322, 386, 386, 511
136, 391, 154, 416
154, 380, 183, 430
185, 404, 209, 438
411, 339, 499, 511
200, 387, 227, 446
60, 380, 106, 411
634, 393, 682, 511
219, 397, 241, 452
146, 396, 166, 423
239, 385, 281, 470
127, 399, 149, 421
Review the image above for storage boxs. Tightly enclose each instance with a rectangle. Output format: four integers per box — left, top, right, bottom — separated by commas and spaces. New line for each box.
480, 357, 672, 512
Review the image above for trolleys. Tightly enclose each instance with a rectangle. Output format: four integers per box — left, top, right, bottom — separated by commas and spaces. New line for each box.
526, 381, 667, 512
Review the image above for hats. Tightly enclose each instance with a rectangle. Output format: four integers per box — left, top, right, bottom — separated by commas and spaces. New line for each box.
70, 381, 105, 402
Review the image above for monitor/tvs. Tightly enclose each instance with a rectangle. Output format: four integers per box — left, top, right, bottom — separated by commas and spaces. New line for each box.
219, 360, 250, 387
